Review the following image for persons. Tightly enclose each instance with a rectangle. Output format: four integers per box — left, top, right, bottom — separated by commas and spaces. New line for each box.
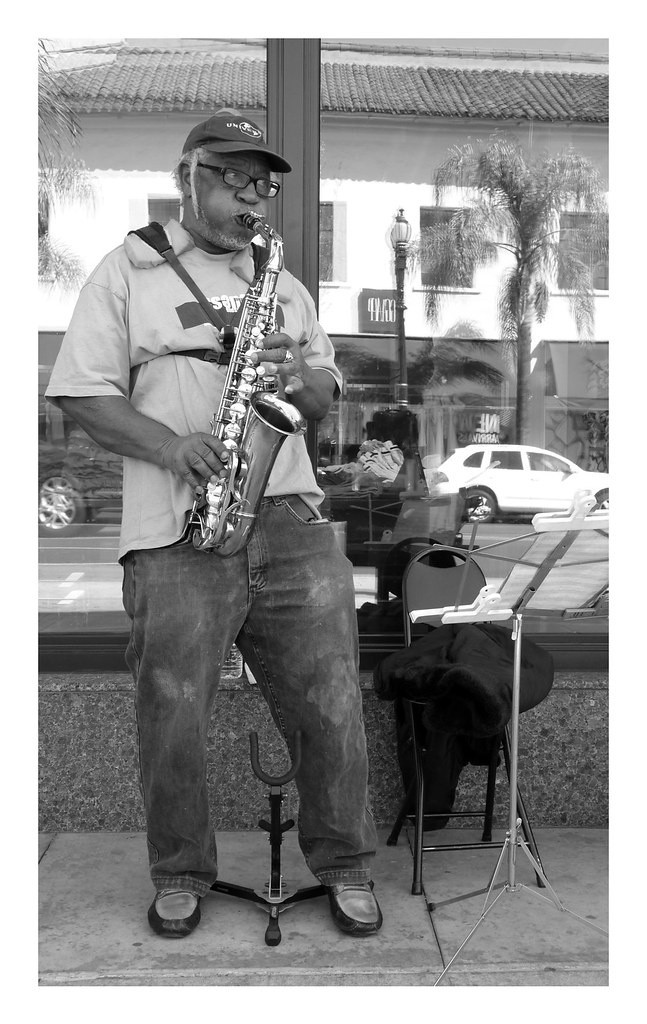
44, 111, 384, 940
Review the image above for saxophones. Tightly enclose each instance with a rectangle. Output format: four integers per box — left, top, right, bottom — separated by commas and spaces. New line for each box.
186, 209, 309, 559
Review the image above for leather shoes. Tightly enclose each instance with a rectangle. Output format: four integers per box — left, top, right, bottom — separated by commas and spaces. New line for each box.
330, 882, 384, 936
147, 889, 200, 938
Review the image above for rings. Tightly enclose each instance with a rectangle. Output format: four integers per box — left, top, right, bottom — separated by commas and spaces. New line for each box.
283, 349, 293, 363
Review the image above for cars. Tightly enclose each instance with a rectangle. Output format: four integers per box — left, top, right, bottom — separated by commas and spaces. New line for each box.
429, 442, 610, 524
39, 440, 125, 539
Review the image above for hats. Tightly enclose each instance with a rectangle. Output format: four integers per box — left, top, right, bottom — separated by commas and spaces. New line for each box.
181, 116, 292, 174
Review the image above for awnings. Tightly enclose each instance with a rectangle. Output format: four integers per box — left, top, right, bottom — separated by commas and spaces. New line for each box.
543, 341, 608, 399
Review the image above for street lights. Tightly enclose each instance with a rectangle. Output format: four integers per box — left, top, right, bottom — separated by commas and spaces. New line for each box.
390, 208, 412, 411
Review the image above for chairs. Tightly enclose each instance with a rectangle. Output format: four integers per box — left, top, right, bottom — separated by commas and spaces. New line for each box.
385, 545, 547, 911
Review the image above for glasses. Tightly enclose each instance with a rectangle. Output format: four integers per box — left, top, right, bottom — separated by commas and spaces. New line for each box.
196, 164, 280, 198
